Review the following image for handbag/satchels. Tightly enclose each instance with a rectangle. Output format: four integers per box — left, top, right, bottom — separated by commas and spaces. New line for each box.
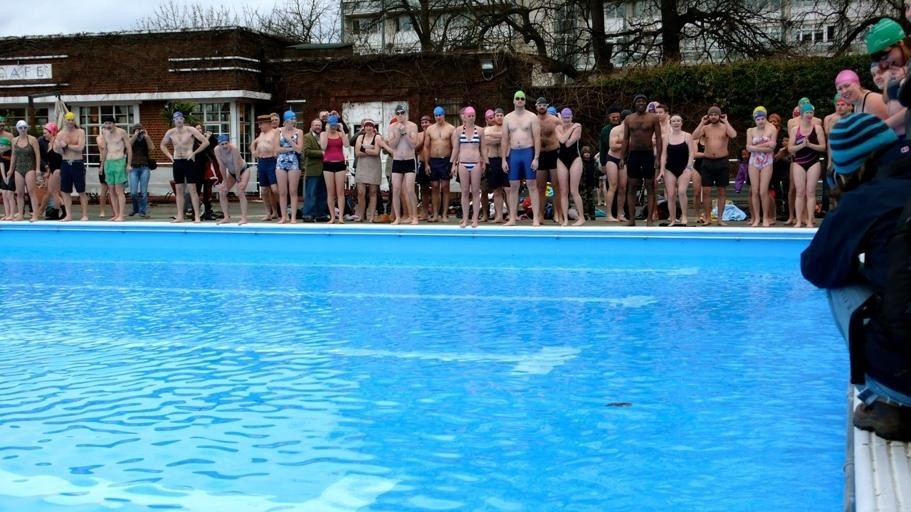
147, 158, 158, 170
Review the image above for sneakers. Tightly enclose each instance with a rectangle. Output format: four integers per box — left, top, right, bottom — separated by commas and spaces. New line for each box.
851, 399, 911, 444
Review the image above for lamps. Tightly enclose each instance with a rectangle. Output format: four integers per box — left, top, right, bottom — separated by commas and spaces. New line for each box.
477, 56, 495, 72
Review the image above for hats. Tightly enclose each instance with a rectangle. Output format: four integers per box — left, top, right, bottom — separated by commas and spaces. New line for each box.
607, 106, 632, 118
829, 111, 899, 176
283, 110, 296, 121
172, 112, 185, 119
869, 61, 880, 69
866, 17, 907, 57
633, 92, 658, 111
791, 97, 817, 118
327, 115, 339, 126
217, 134, 229, 143
707, 106, 722, 116
535, 96, 548, 104
752, 105, 769, 120
548, 106, 573, 117
418, 106, 504, 122
39, 112, 146, 135
15, 119, 29, 128
253, 114, 274, 124
834, 69, 861, 89
833, 94, 852, 107
513, 90, 527, 99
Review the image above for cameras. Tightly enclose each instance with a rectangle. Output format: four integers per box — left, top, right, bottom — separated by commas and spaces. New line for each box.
139, 130, 145, 133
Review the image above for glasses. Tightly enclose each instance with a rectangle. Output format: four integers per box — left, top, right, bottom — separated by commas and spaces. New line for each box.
878, 47, 897, 60
17, 126, 28, 130
514, 96, 525, 101
395, 110, 406, 115
